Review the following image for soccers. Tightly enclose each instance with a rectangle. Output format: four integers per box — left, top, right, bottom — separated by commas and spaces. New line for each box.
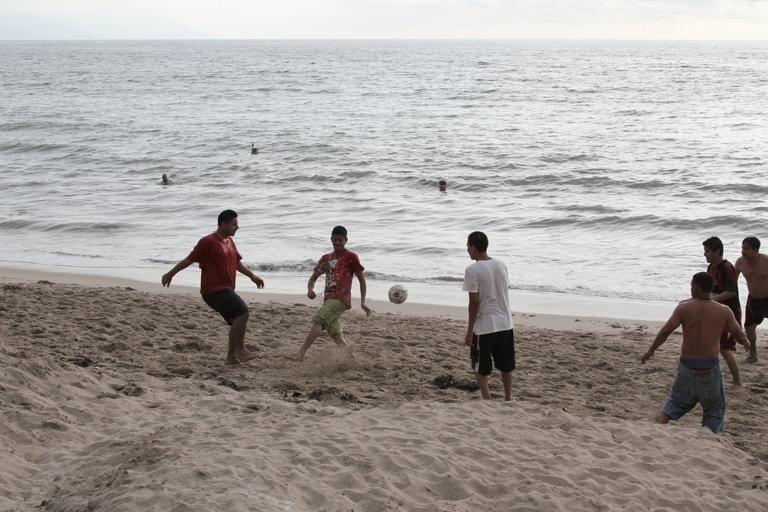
389, 285, 407, 303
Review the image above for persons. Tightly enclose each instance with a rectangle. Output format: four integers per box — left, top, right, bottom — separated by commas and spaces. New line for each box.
700, 235, 743, 389
638, 272, 752, 435
158, 173, 172, 185
281, 224, 372, 363
733, 235, 767, 367
438, 180, 448, 192
161, 209, 264, 367
461, 230, 518, 400
